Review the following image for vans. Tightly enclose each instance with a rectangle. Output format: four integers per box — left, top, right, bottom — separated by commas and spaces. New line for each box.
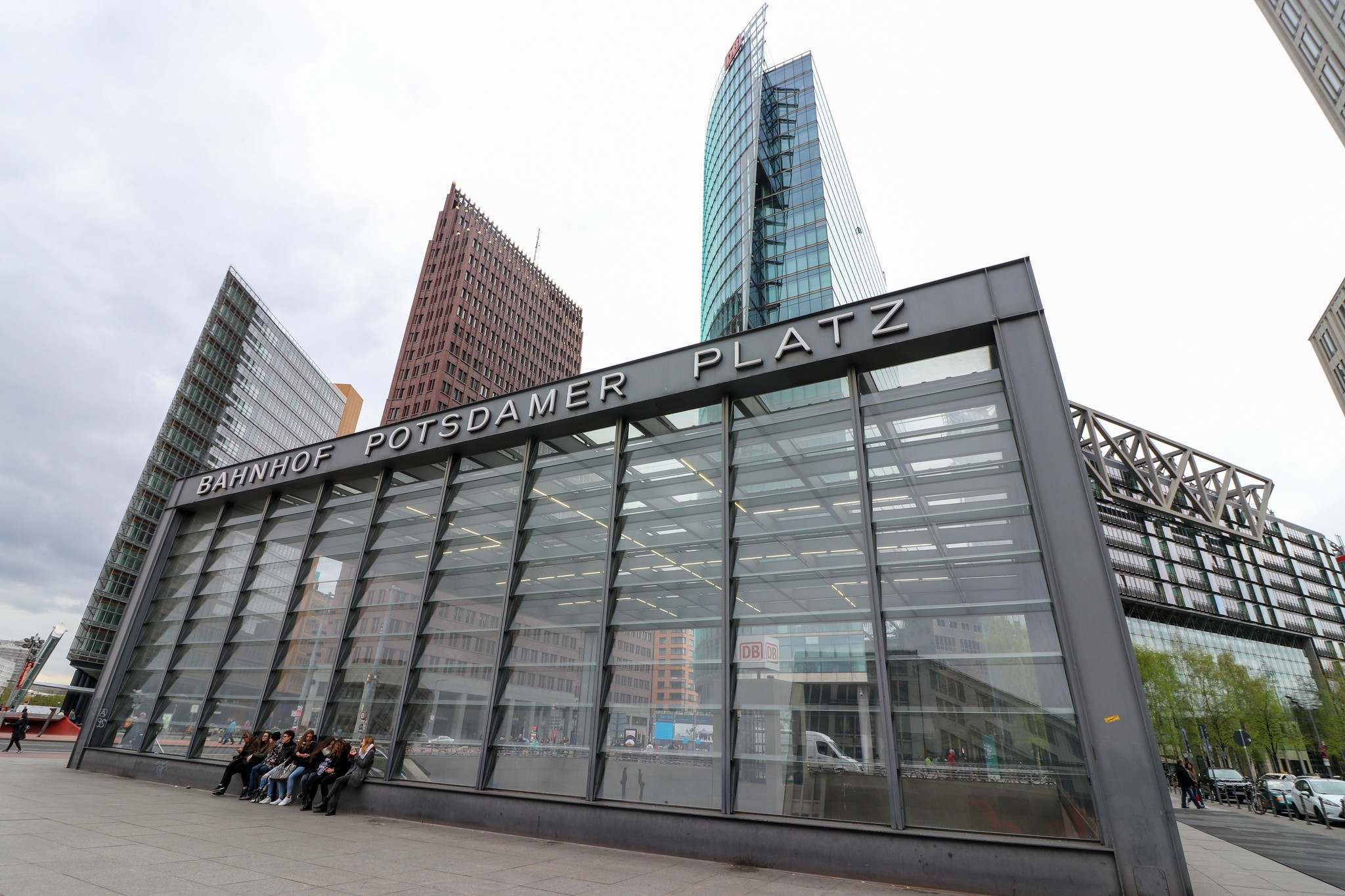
756, 726, 865, 774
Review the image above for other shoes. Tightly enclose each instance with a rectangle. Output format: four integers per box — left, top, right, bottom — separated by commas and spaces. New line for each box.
297, 792, 306, 799
1197, 805, 1205, 809
211, 791, 224, 796
239, 786, 259, 800
1185, 800, 1191, 808
219, 741, 241, 745
1182, 806, 1189, 809
301, 799, 306, 806
212, 785, 225, 792
1203, 805, 1210, 809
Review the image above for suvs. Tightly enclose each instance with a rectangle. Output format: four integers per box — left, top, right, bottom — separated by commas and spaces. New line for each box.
1196, 767, 1252, 803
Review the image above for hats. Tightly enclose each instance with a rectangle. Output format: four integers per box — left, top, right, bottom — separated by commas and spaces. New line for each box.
270, 731, 281, 741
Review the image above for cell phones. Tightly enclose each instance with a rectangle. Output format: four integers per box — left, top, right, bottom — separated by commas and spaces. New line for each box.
299, 754, 306, 757
254, 749, 257, 753
352, 746, 355, 752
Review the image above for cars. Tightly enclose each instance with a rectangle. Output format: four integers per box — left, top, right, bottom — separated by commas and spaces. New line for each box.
407, 732, 429, 746
348, 744, 417, 781
207, 723, 340, 745
428, 735, 455, 748
1290, 776, 1345, 827
1253, 781, 1296, 815
1255, 771, 1345, 782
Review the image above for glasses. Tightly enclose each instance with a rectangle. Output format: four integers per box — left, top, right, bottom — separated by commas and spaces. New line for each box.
262, 735, 268, 737
283, 736, 289, 737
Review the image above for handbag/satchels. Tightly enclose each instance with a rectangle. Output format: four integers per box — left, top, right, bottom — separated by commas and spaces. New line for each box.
122, 742, 132, 749
1196, 794, 1200, 801
269, 759, 297, 781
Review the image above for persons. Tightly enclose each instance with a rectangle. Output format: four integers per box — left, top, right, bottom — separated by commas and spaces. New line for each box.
0, 704, 4, 711
1175, 759, 1211, 809
476, 733, 483, 754
613, 739, 687, 764
1, 712, 28, 754
118, 713, 392, 816
497, 733, 570, 759
923, 749, 972, 778
8, 706, 15, 712
57, 710, 76, 723
21, 707, 28, 713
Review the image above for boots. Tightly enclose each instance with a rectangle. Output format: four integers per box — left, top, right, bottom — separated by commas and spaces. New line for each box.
325, 802, 338, 816
313, 797, 331, 808
313, 803, 328, 813
300, 799, 312, 811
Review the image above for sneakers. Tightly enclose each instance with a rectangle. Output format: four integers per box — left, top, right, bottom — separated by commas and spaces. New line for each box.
278, 795, 292, 806
250, 797, 265, 803
259, 796, 271, 804
1, 750, 8, 753
270, 798, 283, 805
15, 749, 23, 754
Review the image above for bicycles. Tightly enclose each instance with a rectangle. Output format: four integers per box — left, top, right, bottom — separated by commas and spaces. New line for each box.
403, 742, 715, 766
1244, 784, 1268, 815
807, 756, 1054, 785
158, 728, 165, 735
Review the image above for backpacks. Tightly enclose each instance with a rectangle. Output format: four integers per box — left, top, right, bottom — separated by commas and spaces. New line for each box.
22, 724, 30, 733
